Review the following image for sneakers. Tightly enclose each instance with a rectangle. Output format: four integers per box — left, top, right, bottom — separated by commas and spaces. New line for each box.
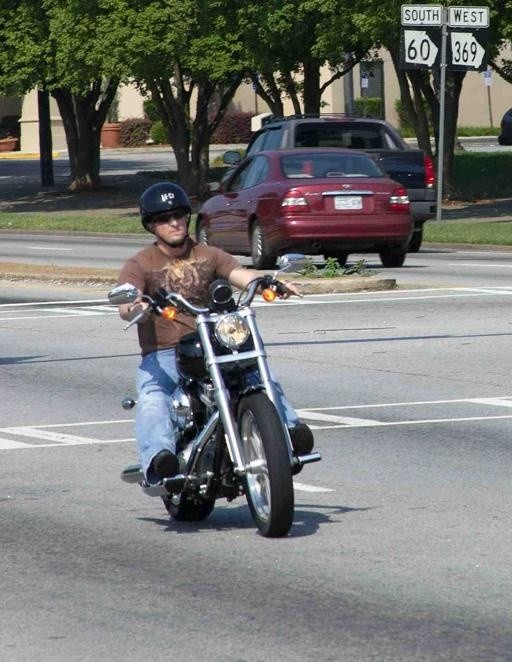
290, 423, 314, 476
153, 450, 184, 495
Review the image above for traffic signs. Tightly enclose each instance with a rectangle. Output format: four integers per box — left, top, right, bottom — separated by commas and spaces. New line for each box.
399, 3, 491, 71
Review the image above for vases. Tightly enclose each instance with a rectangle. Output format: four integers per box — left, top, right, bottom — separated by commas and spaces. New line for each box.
0, 137, 19, 151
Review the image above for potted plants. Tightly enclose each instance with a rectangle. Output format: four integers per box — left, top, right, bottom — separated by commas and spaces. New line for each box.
101, 80, 123, 148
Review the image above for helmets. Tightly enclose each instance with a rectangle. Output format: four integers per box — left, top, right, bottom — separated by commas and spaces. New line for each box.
139, 182, 194, 223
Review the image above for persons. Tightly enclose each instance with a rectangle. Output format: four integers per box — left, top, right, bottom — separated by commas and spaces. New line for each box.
115, 182, 314, 499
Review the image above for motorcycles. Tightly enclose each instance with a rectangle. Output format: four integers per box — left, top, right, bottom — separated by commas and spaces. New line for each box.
107, 274, 323, 538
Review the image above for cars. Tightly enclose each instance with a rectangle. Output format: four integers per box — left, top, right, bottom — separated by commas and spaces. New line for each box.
223, 119, 439, 253
195, 148, 415, 270
